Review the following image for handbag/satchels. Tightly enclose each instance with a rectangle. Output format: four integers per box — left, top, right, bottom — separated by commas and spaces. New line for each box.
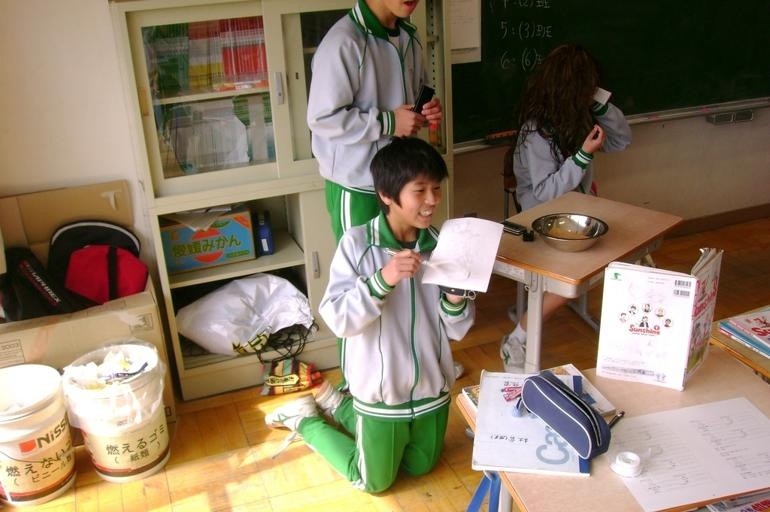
176, 271, 316, 364
64, 245, 148, 308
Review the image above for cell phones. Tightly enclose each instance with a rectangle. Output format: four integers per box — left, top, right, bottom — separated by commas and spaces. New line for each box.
500, 220, 527, 236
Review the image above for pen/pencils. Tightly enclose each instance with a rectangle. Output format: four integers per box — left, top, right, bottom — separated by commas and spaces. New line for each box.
608, 411, 625, 428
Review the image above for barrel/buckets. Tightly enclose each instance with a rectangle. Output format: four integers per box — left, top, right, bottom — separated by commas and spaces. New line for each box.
0, 363, 76, 504
63, 343, 169, 483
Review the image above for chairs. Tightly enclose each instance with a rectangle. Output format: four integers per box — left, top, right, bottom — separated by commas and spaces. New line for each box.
504, 141, 526, 326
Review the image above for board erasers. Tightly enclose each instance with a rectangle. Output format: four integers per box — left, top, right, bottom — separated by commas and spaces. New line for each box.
706, 109, 754, 125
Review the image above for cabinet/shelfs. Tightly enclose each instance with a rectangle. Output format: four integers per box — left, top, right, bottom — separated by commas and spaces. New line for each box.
110, 0, 454, 401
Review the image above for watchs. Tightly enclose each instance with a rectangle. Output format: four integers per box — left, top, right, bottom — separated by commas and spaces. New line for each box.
263, 132, 477, 496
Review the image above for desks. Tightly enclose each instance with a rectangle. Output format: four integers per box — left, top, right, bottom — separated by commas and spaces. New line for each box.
456, 192, 769, 512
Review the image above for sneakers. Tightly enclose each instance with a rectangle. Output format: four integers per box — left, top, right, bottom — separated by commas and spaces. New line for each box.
452, 361, 465, 381
263, 392, 318, 432
314, 380, 345, 421
499, 334, 526, 374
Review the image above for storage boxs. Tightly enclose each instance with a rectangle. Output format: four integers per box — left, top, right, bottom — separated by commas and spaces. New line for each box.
0, 178, 178, 448
159, 208, 258, 276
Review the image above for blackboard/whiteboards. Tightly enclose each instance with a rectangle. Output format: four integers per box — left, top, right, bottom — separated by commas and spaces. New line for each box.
441, 0, 770, 155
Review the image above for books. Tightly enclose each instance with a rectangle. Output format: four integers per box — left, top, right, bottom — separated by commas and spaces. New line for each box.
141, 16, 276, 174
706, 493, 770, 512
470, 368, 592, 478
720, 486, 769, 507
462, 362, 617, 418
702, 304, 769, 383
595, 245, 725, 392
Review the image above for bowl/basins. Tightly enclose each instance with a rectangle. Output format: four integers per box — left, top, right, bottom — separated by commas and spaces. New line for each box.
531, 212, 610, 252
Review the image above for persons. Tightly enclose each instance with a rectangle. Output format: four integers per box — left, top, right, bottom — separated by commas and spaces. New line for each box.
306, 1, 446, 248
497, 43, 633, 375
